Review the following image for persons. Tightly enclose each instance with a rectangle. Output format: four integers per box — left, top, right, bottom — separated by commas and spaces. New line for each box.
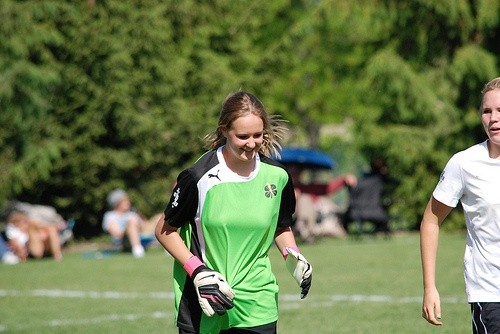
0, 201, 74, 265
155, 91, 313, 334
291, 160, 402, 237
420, 77, 500, 334
100, 189, 175, 258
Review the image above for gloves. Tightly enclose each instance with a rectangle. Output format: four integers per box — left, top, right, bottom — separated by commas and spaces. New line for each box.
190, 264, 235, 317
284, 247, 313, 299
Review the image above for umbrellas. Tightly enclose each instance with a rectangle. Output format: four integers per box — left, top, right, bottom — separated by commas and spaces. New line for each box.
268, 146, 334, 169
